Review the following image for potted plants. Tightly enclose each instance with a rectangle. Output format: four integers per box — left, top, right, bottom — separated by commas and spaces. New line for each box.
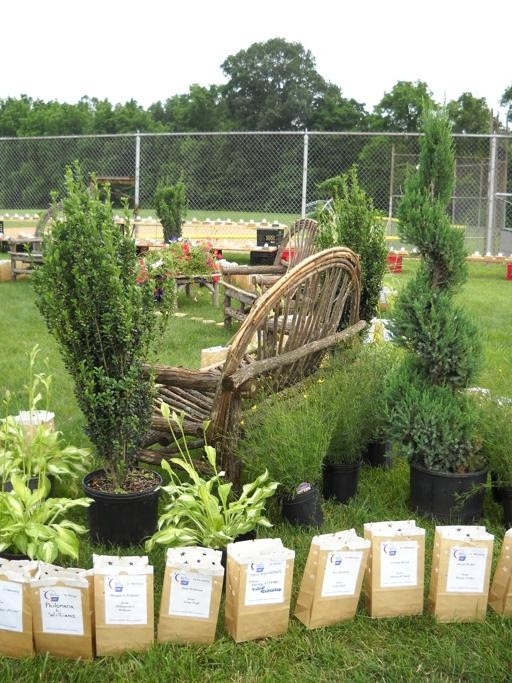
0, 158, 512, 561
